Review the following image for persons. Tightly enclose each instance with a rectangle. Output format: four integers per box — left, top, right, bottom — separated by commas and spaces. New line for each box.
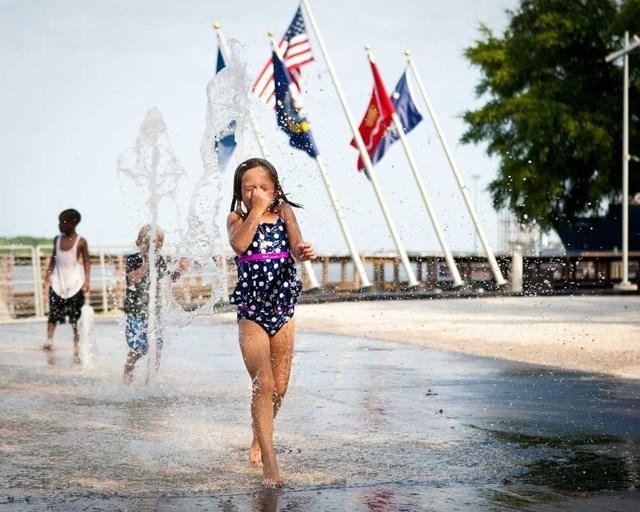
123, 223, 189, 384
224, 158, 317, 488
40, 208, 91, 358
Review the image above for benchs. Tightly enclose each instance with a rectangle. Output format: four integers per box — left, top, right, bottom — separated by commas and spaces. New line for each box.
252, 5, 314, 117
346, 50, 396, 171
364, 67, 428, 183
204, 36, 240, 176
271, 41, 318, 158
